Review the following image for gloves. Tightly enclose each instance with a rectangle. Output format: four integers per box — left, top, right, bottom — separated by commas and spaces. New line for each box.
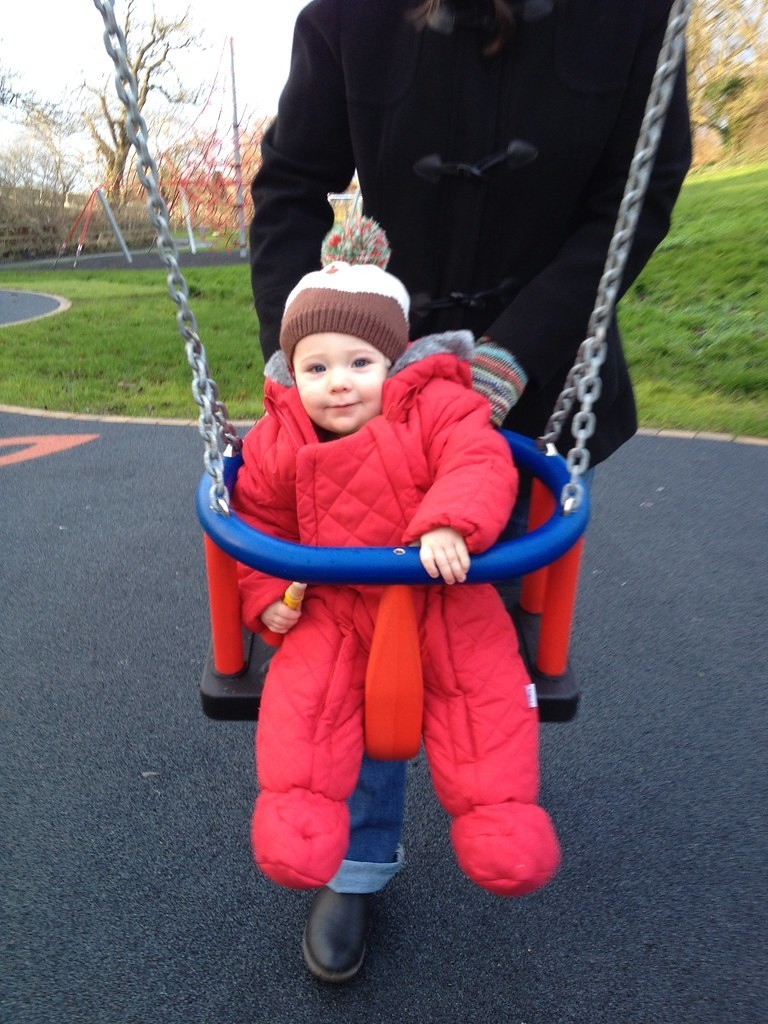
463, 340, 528, 430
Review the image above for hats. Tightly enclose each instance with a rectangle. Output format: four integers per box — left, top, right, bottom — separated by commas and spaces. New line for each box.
279, 221, 412, 365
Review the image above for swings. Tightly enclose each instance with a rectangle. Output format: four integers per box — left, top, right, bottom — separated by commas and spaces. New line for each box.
86, 1, 692, 726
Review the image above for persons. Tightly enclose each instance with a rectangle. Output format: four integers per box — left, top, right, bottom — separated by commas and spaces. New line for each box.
249, 0, 692, 983
229, 215, 561, 897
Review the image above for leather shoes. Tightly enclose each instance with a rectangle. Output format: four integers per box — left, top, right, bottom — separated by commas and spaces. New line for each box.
302, 884, 373, 980
